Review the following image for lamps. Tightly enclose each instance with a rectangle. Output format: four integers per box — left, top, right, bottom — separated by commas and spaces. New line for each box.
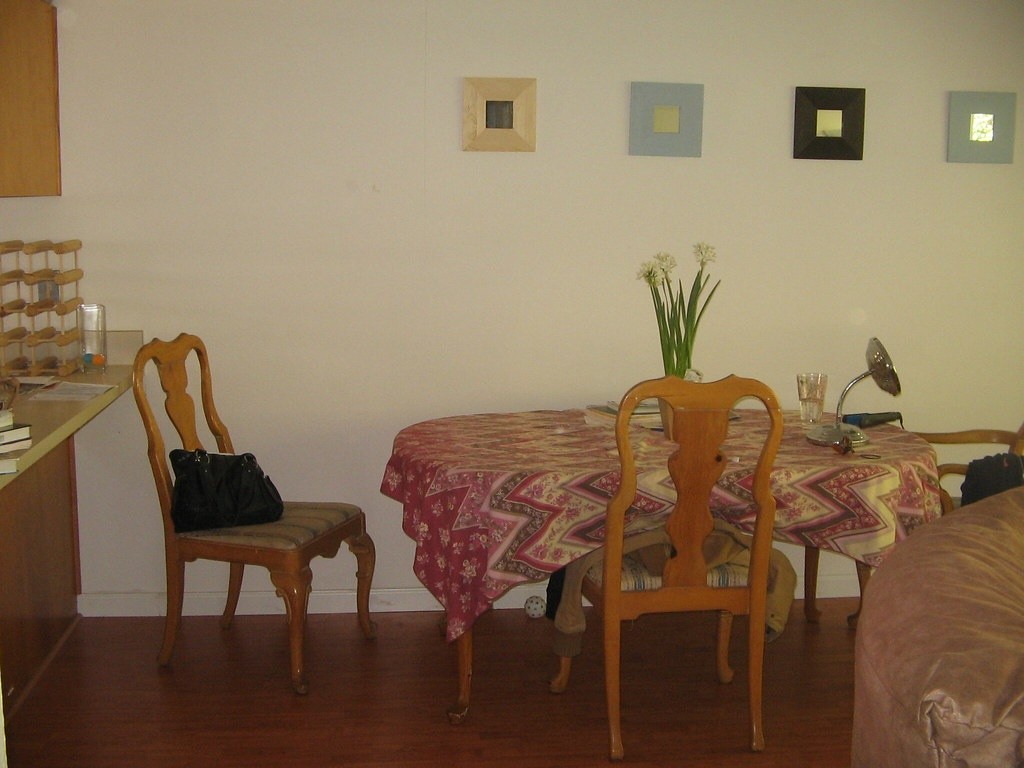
805, 338, 900, 447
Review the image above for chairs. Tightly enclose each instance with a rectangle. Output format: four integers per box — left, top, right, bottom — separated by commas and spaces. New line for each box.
550, 374, 783, 761
133, 331, 378, 694
846, 425, 1024, 629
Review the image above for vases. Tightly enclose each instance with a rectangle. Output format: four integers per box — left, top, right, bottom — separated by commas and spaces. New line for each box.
657, 398, 674, 441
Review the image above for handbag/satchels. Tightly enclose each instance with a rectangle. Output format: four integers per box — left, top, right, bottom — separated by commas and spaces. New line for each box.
169, 448, 284, 532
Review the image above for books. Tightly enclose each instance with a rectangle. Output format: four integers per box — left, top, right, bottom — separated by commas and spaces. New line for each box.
0, 410, 32, 474
584, 399, 740, 428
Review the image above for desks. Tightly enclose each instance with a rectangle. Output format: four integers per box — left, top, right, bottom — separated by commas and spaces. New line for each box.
379, 408, 938, 725
0, 330, 145, 720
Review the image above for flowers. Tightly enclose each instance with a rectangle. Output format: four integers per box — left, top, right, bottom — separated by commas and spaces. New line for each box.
634, 240, 722, 377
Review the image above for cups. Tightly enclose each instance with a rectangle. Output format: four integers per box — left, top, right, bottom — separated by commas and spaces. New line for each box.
796, 374, 828, 427
77, 304, 107, 375
